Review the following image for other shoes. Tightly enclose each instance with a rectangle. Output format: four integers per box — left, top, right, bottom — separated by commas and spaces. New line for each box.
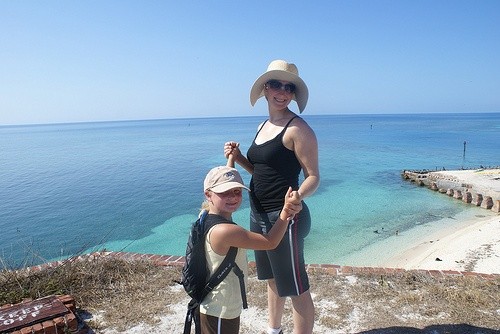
261, 330, 283, 334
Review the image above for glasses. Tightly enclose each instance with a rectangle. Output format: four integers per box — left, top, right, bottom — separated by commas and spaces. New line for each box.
264, 80, 296, 93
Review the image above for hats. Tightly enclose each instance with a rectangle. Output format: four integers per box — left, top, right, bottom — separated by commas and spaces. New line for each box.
204, 166, 251, 193
250, 60, 309, 114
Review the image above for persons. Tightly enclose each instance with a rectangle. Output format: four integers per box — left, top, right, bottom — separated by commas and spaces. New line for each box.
224, 59, 320, 334
197, 141, 301, 334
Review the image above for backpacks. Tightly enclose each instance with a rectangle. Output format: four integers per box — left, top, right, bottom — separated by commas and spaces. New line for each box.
182, 209, 248, 334
